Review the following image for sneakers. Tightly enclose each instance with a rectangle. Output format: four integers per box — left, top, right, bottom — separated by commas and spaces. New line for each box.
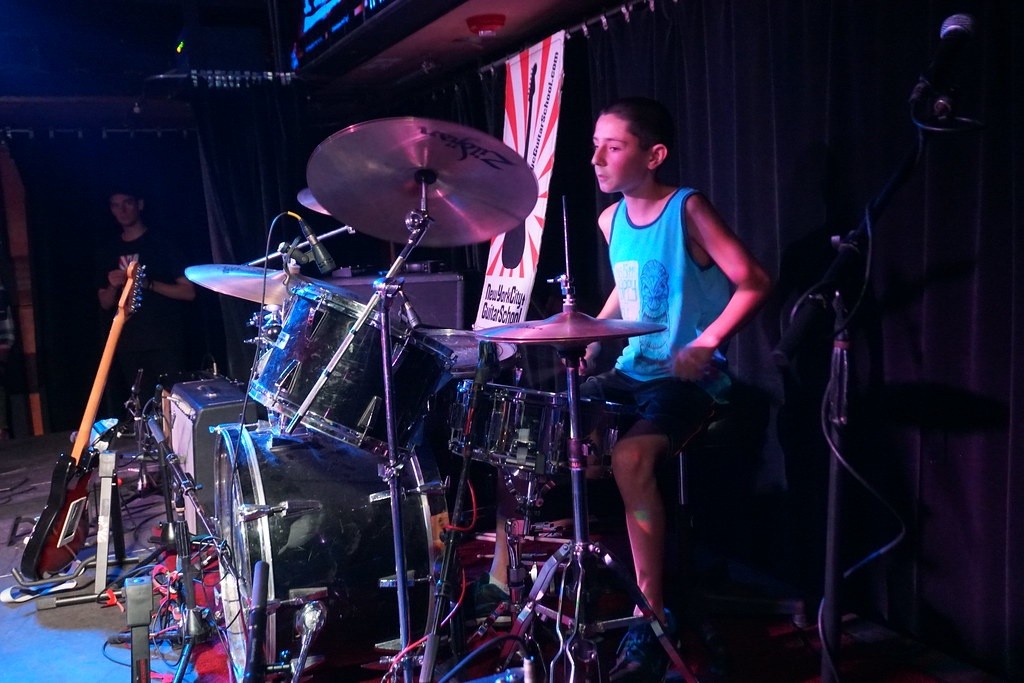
610, 609, 681, 682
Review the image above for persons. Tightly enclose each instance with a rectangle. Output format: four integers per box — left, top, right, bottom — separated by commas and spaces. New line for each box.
559, 98, 776, 683
96, 186, 196, 397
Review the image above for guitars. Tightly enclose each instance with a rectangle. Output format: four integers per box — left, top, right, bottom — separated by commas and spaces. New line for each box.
20, 260, 153, 583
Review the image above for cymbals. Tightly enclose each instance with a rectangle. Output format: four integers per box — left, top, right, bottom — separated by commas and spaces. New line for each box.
474, 309, 670, 350
296, 188, 332, 216
183, 260, 371, 313
305, 116, 538, 248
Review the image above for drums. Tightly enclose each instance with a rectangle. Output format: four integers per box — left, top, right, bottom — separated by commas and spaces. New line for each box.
209, 423, 438, 683
447, 376, 626, 477
407, 326, 519, 447
247, 278, 457, 466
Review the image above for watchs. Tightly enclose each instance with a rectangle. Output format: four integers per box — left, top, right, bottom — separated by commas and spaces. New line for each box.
146, 278, 154, 292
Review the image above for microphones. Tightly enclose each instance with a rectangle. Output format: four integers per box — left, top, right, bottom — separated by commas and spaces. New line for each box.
243, 561, 271, 683
933, 12, 973, 119
297, 215, 337, 276
